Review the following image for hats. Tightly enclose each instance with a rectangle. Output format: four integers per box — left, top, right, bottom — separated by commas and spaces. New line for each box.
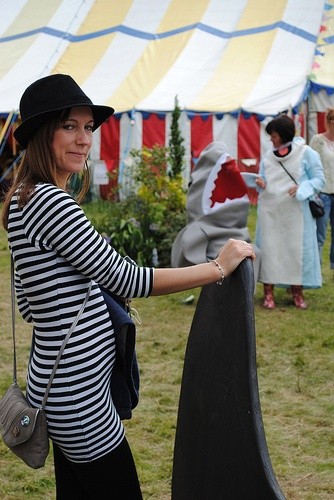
12, 74, 115, 150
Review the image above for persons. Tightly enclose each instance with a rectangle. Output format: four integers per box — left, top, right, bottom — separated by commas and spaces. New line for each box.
1, 73, 258, 499
252, 115, 325, 310
305, 109, 334, 268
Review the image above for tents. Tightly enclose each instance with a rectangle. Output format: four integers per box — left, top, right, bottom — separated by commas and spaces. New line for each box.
0, 0, 334, 205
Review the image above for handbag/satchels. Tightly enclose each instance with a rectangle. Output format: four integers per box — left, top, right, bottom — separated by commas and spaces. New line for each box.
309, 200, 325, 218
0, 383, 49, 471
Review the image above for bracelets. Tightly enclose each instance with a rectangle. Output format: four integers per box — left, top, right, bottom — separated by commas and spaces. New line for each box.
209, 260, 226, 285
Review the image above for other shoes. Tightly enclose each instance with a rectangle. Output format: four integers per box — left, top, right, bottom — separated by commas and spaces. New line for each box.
261, 283, 277, 309
292, 285, 308, 309
